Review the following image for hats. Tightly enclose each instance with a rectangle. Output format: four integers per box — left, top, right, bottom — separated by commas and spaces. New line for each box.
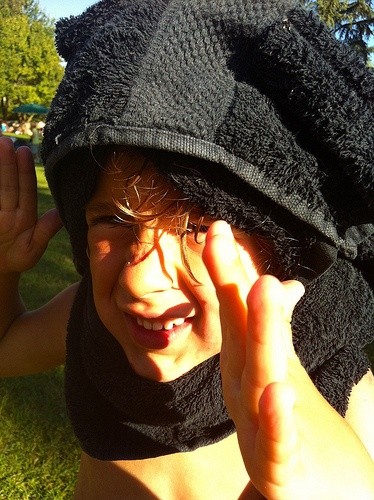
43, 0, 373, 460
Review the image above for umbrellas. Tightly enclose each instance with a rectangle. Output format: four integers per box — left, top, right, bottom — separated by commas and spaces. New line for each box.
11, 103, 48, 114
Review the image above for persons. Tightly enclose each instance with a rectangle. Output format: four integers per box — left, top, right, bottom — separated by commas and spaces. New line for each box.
0, 0, 374, 500
0, 114, 44, 143
